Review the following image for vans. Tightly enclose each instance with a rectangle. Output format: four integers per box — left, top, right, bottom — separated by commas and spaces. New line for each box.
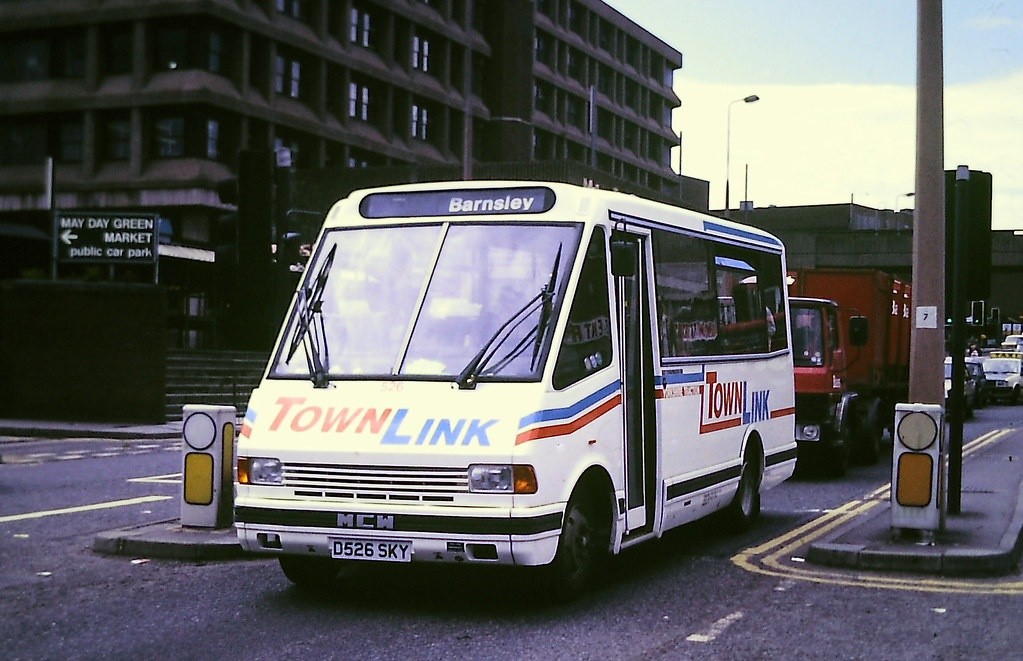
230, 179, 798, 612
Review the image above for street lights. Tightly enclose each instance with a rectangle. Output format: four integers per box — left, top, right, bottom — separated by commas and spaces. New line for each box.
725, 94, 761, 221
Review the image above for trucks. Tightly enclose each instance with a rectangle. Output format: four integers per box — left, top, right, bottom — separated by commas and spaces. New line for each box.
720, 264, 908, 479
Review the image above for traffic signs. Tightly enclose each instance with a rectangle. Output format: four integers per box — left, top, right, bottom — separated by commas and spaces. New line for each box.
53, 210, 158, 265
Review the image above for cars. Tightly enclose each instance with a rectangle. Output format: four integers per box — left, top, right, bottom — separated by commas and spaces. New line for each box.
941, 333, 1023, 423
